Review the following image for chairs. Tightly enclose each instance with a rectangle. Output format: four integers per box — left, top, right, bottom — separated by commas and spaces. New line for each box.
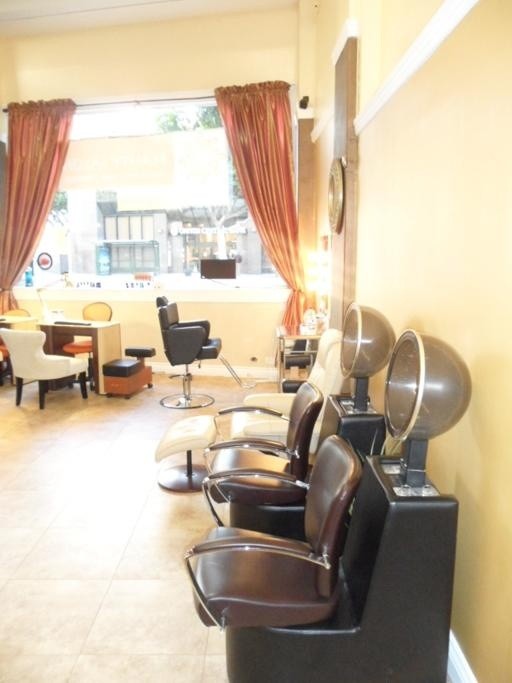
203, 301, 397, 528
183, 329, 473, 683
156, 298, 256, 408
155, 329, 346, 492
0, 301, 112, 409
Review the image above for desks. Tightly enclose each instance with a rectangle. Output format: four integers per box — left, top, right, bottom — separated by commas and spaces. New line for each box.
38, 318, 123, 394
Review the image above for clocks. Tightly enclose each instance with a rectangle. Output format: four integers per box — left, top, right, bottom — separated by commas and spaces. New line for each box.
327, 158, 343, 233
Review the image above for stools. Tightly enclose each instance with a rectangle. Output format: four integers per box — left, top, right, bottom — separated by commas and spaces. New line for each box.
123, 346, 156, 365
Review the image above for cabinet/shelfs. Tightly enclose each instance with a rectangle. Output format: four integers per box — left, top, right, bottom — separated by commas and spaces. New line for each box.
277, 332, 320, 391
